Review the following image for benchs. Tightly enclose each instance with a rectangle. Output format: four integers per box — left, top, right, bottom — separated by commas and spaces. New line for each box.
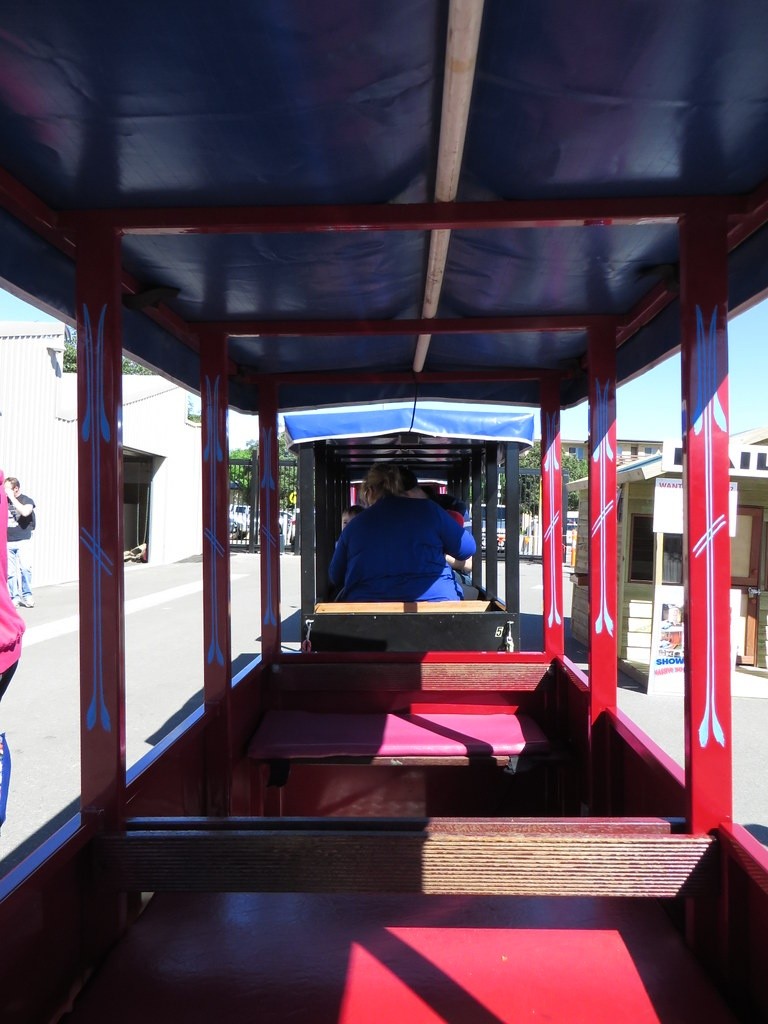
65, 826, 768, 1024
240, 662, 573, 817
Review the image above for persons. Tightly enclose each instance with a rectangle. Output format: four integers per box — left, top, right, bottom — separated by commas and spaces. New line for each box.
399, 467, 473, 586
329, 462, 477, 602
341, 504, 363, 530
0, 463, 26, 826
5, 477, 36, 609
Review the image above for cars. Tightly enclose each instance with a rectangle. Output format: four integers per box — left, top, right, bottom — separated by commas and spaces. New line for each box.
479, 504, 507, 553
